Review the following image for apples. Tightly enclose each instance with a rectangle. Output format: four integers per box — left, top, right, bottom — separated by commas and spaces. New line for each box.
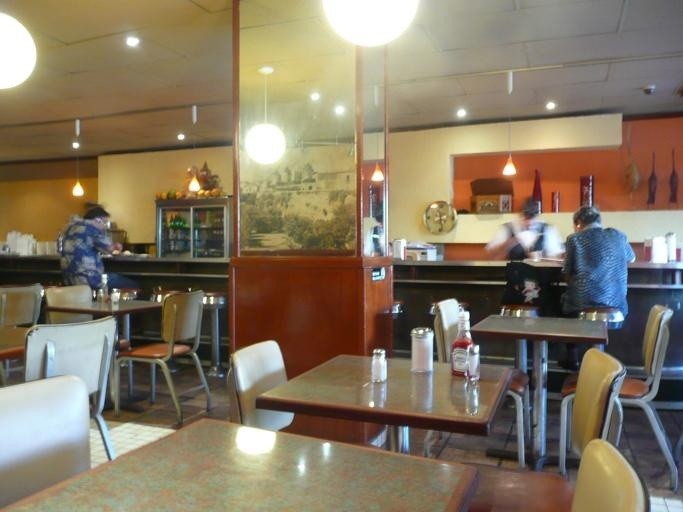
204, 191, 211, 198
213, 189, 220, 196
176, 192, 181, 200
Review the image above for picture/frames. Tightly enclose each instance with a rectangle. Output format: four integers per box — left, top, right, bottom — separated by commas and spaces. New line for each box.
232, 1, 359, 257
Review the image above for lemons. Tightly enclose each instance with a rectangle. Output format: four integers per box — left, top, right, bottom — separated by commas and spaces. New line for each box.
161, 192, 167, 200
199, 190, 205, 198
168, 191, 176, 200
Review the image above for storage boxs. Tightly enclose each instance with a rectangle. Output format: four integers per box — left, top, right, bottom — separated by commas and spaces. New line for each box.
466, 177, 515, 215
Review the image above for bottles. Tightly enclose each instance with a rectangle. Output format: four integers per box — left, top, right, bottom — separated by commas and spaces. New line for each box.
371, 383, 386, 406
166, 213, 187, 226
531, 169, 542, 214
87, 272, 120, 305
464, 381, 479, 414
193, 209, 223, 226
449, 311, 474, 377
167, 228, 188, 239
168, 241, 189, 256
195, 230, 223, 241
448, 377, 467, 416
411, 373, 432, 412
409, 327, 434, 372
645, 151, 657, 209
667, 148, 678, 209
464, 346, 479, 380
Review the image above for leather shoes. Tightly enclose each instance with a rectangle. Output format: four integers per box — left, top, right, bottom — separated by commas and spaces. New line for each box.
570, 360, 580, 369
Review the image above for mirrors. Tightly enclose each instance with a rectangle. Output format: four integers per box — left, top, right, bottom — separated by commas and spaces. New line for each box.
360, 39, 387, 258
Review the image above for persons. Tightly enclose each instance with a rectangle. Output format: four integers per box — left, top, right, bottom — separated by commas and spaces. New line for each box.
55, 205, 144, 336
552, 206, 638, 372
483, 195, 564, 261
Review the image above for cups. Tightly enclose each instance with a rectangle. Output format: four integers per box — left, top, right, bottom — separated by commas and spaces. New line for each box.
370, 350, 386, 382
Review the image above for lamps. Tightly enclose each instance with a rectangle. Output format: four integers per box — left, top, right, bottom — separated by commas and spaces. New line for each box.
501, 70, 518, 176
187, 103, 204, 193
243, 66, 289, 166
68, 117, 88, 197
369, 84, 384, 183
